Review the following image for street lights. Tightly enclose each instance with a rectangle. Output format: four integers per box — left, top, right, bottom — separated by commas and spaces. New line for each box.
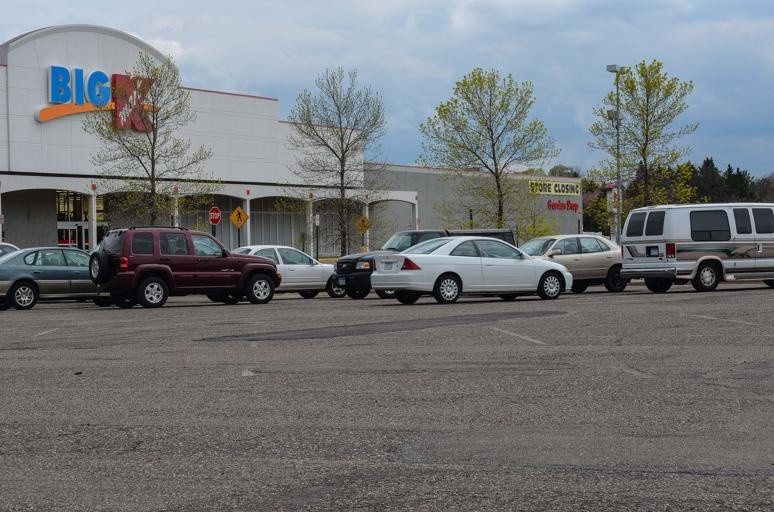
607, 64, 632, 240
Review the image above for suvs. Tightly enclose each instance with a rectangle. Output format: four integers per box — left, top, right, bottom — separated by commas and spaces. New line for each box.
89, 227, 281, 307
331, 230, 518, 300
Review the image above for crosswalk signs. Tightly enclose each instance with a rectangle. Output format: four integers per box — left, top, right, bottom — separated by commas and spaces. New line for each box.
356, 215, 369, 234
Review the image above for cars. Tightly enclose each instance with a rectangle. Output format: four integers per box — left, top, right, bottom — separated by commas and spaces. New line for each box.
228, 245, 345, 298
371, 234, 572, 303
518, 236, 630, 293
0, 242, 112, 310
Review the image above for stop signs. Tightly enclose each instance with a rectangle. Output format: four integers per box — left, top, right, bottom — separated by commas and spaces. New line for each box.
209, 207, 221, 225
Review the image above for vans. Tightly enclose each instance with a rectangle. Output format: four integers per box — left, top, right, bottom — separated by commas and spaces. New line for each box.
618, 200, 774, 292
